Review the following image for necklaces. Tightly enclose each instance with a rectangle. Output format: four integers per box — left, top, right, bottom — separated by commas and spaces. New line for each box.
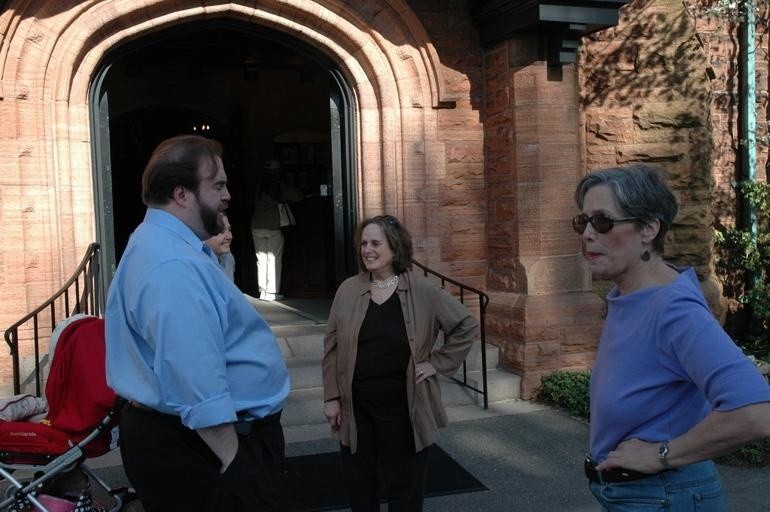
373, 276, 399, 289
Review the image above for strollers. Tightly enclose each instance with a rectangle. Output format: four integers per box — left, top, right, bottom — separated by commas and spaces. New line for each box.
0, 311, 139, 512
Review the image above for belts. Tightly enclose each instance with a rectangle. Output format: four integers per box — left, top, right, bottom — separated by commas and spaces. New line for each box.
583, 452, 673, 487
123, 394, 153, 414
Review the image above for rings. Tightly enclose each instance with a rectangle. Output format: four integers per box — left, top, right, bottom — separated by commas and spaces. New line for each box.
421, 370, 423, 374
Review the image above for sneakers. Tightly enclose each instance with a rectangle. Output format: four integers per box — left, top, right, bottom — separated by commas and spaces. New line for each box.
259, 292, 285, 302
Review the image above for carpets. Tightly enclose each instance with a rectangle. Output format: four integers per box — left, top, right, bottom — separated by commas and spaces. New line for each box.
259, 442, 490, 512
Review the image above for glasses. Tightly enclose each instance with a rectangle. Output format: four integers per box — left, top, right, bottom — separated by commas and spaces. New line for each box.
372, 215, 401, 225
572, 212, 642, 234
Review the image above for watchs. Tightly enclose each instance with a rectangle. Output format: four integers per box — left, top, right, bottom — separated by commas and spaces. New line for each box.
658, 441, 671, 470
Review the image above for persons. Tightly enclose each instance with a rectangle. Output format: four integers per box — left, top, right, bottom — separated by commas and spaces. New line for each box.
204, 211, 236, 285
105, 135, 292, 512
250, 159, 305, 301
572, 164, 770, 512
321, 215, 478, 512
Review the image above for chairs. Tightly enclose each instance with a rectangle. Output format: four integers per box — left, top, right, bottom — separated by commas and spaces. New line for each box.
0, 318, 120, 511
